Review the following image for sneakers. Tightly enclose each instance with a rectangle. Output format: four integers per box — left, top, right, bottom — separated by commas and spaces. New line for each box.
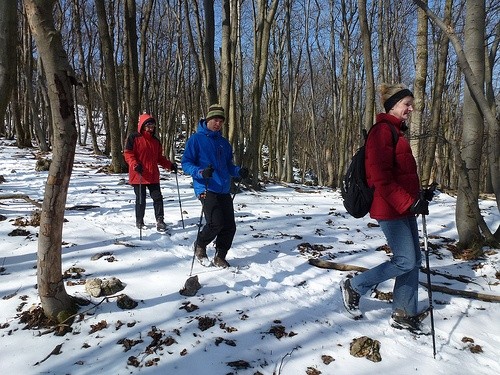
389, 312, 431, 335
340, 279, 363, 319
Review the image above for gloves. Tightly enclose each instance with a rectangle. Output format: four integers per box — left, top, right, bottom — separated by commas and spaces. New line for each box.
171, 163, 178, 174
238, 168, 249, 178
410, 198, 429, 215
202, 164, 214, 177
134, 164, 143, 174
422, 189, 435, 201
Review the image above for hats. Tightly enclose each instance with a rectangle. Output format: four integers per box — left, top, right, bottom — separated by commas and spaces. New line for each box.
144, 120, 155, 127
205, 104, 225, 122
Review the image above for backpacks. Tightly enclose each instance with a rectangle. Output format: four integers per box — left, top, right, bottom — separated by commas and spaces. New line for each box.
341, 120, 395, 218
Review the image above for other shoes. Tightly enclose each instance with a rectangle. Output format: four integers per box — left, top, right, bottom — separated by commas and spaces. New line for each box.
214, 254, 230, 269
136, 222, 147, 230
194, 247, 212, 267
157, 221, 169, 232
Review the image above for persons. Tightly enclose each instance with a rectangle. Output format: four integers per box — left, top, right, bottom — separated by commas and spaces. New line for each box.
181, 104, 249, 268
341, 83, 435, 335
124, 115, 177, 228
